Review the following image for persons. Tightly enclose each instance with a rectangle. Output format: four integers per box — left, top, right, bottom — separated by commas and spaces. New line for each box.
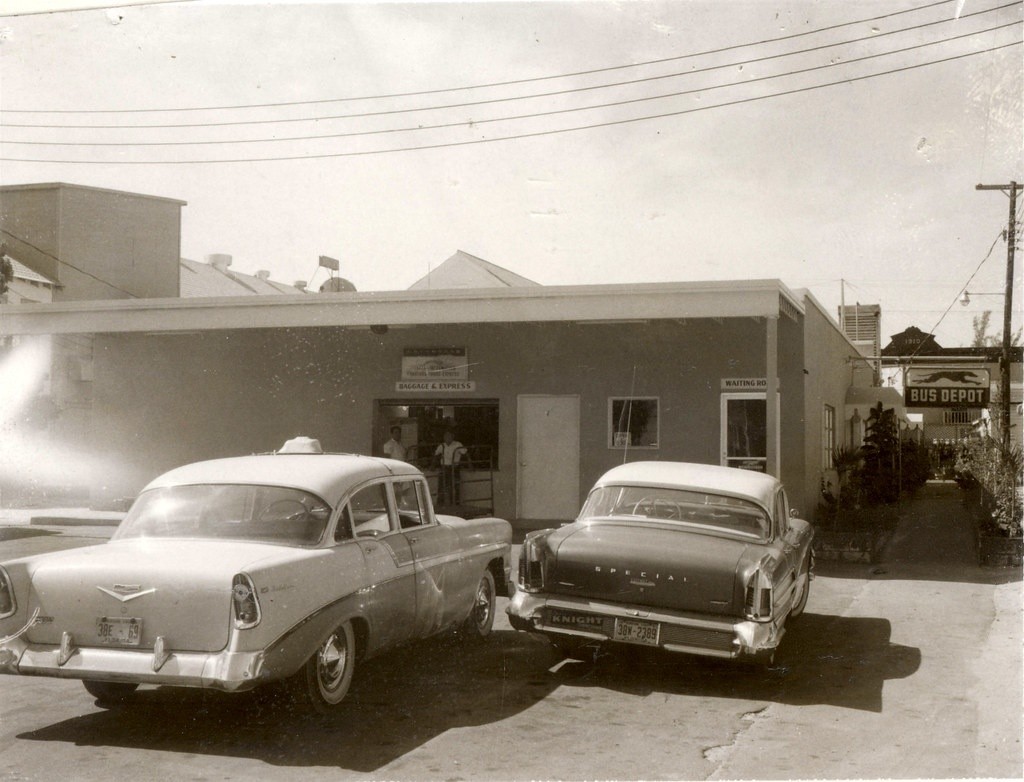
429, 429, 473, 506
383, 425, 407, 462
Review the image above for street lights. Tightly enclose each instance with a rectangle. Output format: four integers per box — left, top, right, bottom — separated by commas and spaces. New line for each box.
959, 291, 1013, 456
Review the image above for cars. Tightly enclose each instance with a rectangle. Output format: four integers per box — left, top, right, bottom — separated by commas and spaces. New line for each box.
0, 437, 518, 717
504, 461, 819, 686
